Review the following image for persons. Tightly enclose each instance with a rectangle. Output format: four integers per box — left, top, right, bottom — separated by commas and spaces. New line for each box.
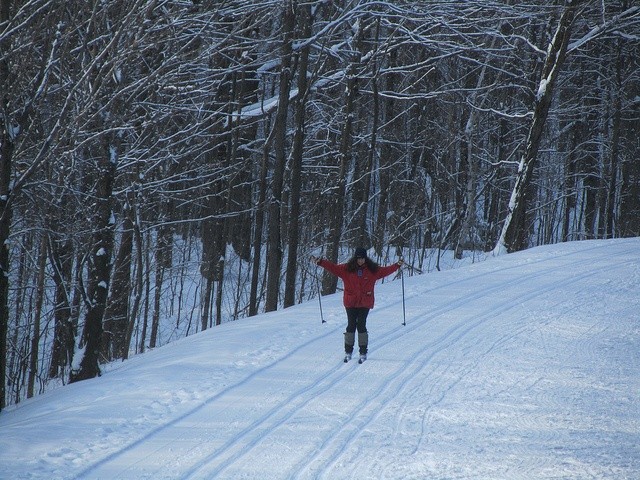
308, 244, 402, 364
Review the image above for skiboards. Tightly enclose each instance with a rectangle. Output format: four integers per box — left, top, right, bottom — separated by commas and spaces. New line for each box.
343, 356, 367, 364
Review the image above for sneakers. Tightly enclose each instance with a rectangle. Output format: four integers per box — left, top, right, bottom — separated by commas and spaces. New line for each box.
358, 355, 367, 363
344, 354, 351, 363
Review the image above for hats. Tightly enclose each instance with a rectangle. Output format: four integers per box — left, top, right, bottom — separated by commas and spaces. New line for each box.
355, 249, 367, 258
